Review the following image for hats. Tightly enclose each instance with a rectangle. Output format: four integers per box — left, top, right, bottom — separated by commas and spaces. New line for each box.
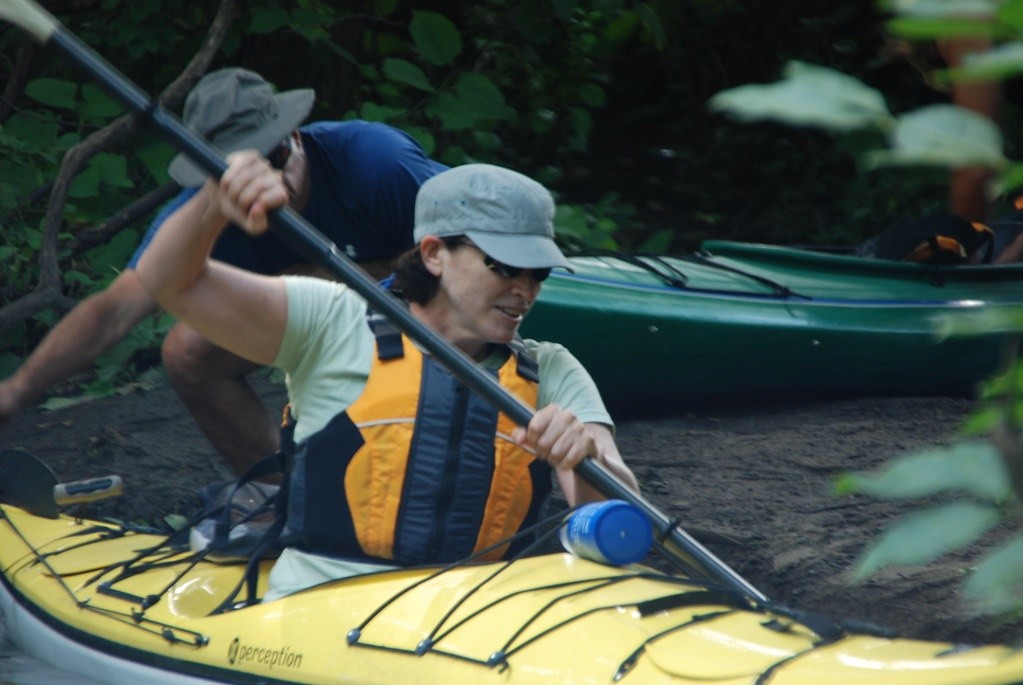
412, 164, 578, 280
168, 67, 316, 187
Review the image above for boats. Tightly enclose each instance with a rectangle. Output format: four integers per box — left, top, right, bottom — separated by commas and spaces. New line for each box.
520, 240, 1022, 402
0, 443, 1022, 685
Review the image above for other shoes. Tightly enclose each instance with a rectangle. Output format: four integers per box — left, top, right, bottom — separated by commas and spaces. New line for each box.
188, 473, 296, 563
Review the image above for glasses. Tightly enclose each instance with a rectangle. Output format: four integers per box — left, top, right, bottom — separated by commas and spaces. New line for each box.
451, 239, 551, 285
261, 131, 292, 169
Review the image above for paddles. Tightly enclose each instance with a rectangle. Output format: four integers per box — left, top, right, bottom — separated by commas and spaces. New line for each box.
1, 0, 771, 610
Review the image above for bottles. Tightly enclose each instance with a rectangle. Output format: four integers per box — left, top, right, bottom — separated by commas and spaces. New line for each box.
558, 499, 654, 565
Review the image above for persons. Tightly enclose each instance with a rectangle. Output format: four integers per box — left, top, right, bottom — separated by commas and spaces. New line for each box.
992, 197, 1023, 263
135, 146, 640, 607
0, 66, 458, 564
